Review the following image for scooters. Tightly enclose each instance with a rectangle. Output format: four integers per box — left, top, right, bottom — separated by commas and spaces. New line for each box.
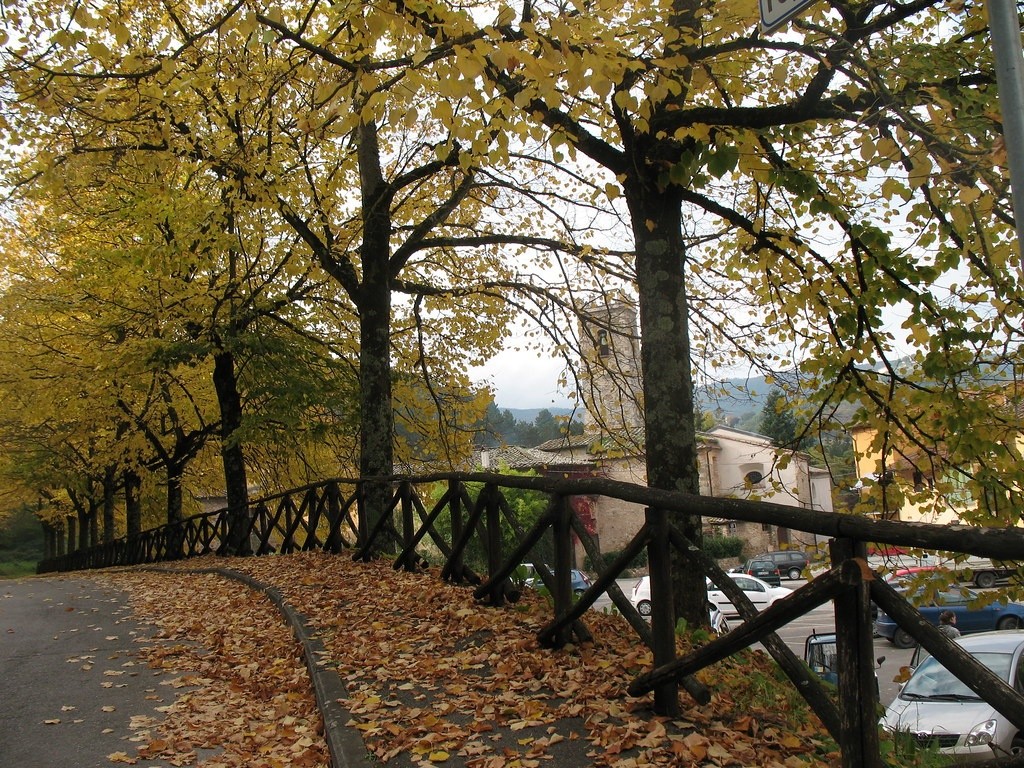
805, 633, 885, 705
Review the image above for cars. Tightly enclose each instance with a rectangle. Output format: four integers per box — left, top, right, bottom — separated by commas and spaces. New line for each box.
876, 583, 1024, 648
631, 577, 652, 616
740, 559, 781, 587
534, 571, 592, 600
707, 574, 794, 615
877, 626, 1024, 767
708, 601, 731, 637
882, 566, 956, 586
520, 563, 554, 588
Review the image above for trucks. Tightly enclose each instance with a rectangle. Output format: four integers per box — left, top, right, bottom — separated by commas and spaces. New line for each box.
936, 548, 1024, 588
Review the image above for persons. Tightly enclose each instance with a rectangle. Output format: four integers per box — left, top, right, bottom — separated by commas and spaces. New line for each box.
938, 609, 961, 640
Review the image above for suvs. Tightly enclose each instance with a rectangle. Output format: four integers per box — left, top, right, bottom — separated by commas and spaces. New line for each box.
727, 551, 810, 580
